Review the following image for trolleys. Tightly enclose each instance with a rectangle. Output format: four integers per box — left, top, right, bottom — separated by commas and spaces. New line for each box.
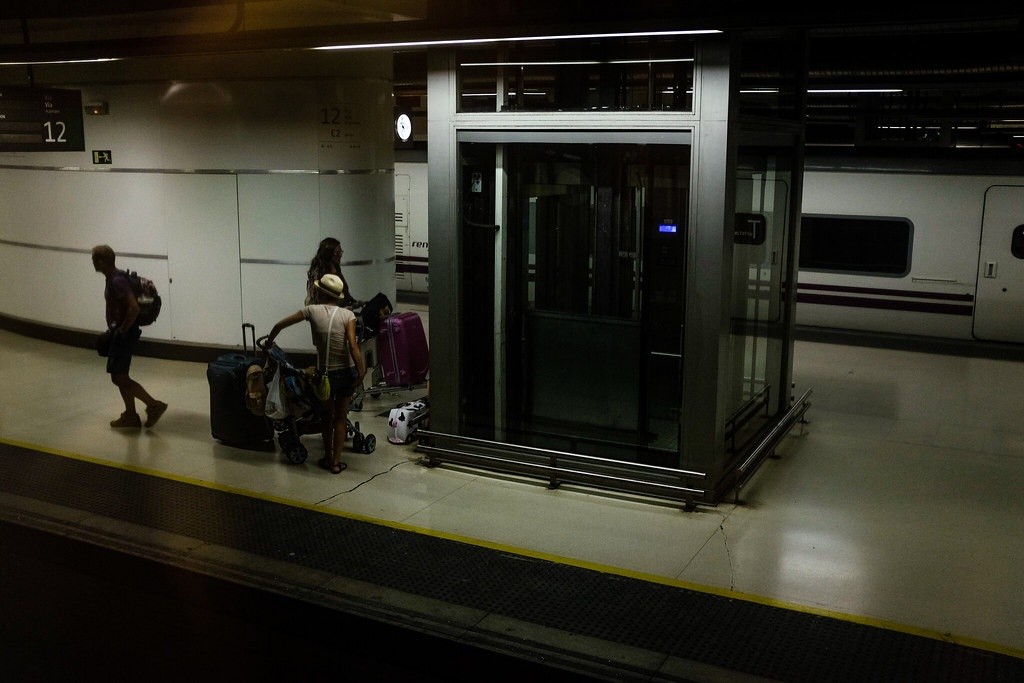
342, 300, 431, 414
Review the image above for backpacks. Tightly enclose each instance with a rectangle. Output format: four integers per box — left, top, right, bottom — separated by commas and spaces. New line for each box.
354, 292, 394, 341
107, 268, 162, 327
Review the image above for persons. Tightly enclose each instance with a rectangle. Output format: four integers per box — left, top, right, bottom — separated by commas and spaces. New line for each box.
264, 276, 367, 475
305, 238, 369, 367
92, 243, 167, 430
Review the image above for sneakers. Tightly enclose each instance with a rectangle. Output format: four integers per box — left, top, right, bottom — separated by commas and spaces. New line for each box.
144, 401, 168, 428
109, 411, 142, 428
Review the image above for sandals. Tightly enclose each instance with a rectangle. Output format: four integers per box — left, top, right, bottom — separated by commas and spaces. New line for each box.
331, 461, 342, 475
319, 457, 332, 469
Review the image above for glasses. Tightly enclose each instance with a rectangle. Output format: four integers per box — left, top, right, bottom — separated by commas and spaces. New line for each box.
333, 251, 343, 257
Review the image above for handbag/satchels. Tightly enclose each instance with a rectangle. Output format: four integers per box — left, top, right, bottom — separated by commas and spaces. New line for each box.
312, 369, 330, 401
264, 363, 291, 420
96, 329, 113, 357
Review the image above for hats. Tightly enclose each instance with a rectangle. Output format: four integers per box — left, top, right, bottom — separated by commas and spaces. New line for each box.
313, 273, 345, 300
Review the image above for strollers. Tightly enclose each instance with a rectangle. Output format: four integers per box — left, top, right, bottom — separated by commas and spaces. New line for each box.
257, 333, 377, 466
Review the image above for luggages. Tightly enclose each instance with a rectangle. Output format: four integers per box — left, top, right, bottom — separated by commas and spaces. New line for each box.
206, 323, 275, 448
359, 338, 379, 377
378, 311, 428, 391
